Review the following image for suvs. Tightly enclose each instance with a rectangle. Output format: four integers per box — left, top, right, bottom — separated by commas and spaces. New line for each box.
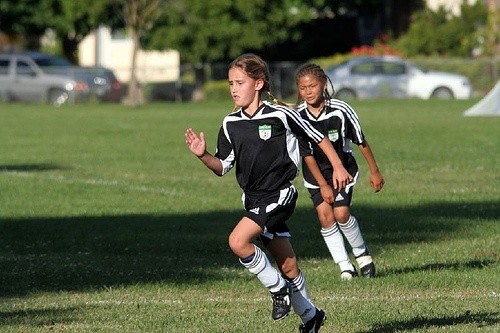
0, 51, 117, 108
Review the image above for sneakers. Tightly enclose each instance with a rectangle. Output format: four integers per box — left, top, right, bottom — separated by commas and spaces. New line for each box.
270, 279, 293, 322
339, 265, 358, 280
355, 245, 375, 276
299, 307, 327, 333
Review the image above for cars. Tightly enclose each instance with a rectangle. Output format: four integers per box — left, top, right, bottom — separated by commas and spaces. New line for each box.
325, 56, 472, 103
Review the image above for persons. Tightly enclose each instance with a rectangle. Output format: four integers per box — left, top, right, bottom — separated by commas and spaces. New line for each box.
184, 53, 354, 333
292, 63, 386, 282
